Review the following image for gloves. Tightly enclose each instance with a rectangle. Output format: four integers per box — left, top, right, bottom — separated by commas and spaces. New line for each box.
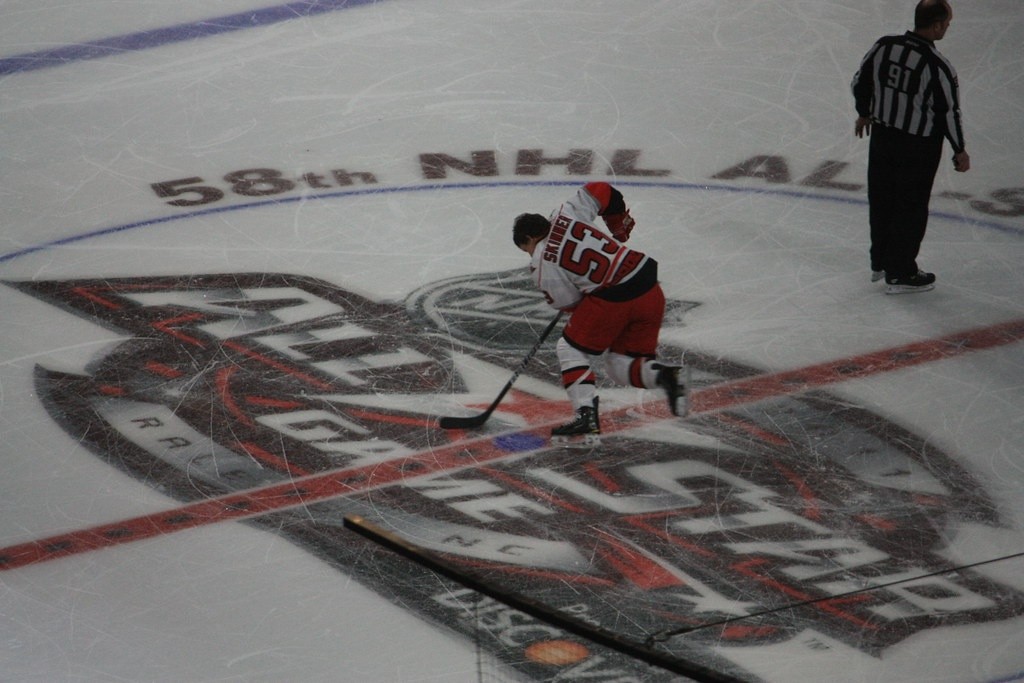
952, 150, 970, 173
602, 207, 635, 243
855, 117, 872, 138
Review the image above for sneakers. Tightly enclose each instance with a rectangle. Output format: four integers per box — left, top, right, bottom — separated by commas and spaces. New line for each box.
549, 395, 602, 448
651, 364, 688, 417
872, 266, 936, 294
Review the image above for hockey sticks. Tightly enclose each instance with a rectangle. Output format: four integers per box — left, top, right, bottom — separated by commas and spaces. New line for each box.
438, 234, 617, 428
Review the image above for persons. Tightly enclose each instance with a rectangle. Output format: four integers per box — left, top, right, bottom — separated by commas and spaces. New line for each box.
850, 0, 970, 294
514, 181, 690, 448
